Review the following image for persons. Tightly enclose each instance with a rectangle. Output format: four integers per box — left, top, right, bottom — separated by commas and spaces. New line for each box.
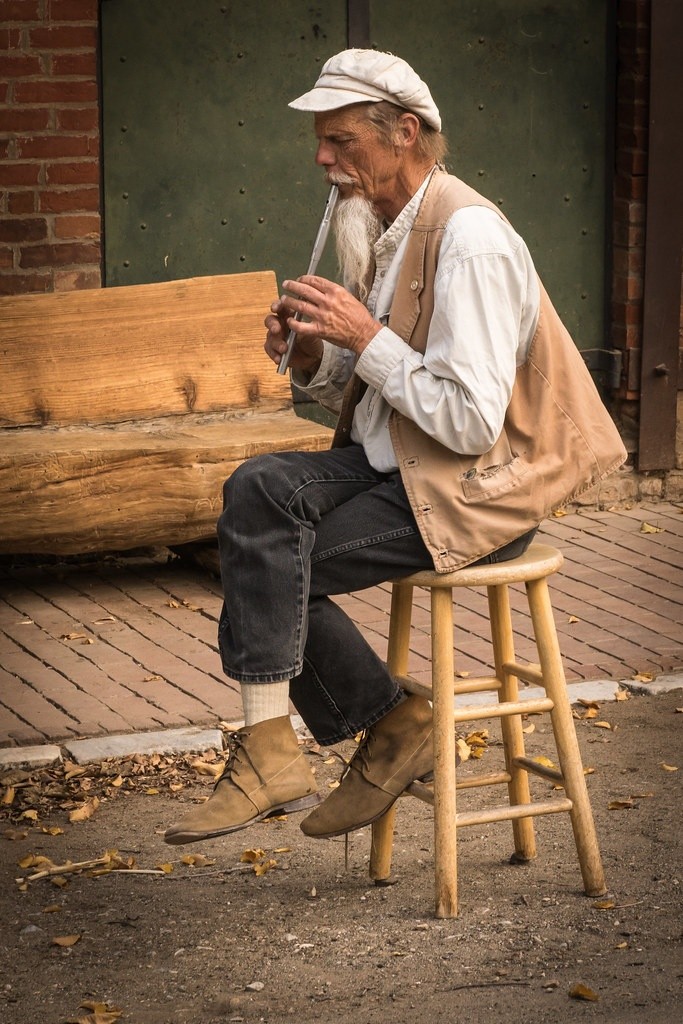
162, 47, 627, 848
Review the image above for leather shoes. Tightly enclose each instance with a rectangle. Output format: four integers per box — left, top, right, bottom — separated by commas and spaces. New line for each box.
163, 717, 320, 844
301, 694, 460, 839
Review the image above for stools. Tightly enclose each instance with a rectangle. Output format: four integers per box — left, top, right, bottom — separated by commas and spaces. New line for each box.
366, 543, 607, 919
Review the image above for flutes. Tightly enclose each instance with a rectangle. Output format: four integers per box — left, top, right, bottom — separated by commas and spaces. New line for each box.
276, 184, 340, 377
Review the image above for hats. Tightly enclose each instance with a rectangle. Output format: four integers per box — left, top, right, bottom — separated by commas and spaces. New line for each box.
288, 47, 442, 131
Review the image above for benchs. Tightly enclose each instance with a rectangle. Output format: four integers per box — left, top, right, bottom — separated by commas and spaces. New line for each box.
0, 269, 338, 554
